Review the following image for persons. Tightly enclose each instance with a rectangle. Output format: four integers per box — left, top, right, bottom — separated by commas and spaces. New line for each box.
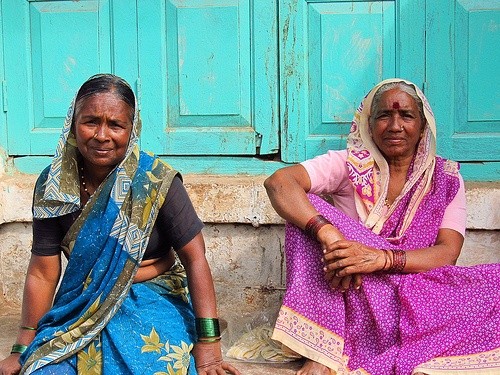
264, 79, 500, 375
0, 74, 241, 375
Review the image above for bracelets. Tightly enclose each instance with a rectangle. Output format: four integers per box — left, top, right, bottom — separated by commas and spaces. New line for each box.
305, 214, 334, 240
21, 327, 37, 329
196, 318, 220, 337
11, 344, 27, 353
197, 338, 221, 343
380, 248, 406, 273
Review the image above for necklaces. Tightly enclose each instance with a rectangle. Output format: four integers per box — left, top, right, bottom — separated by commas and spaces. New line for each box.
385, 198, 390, 209
81, 166, 91, 196
